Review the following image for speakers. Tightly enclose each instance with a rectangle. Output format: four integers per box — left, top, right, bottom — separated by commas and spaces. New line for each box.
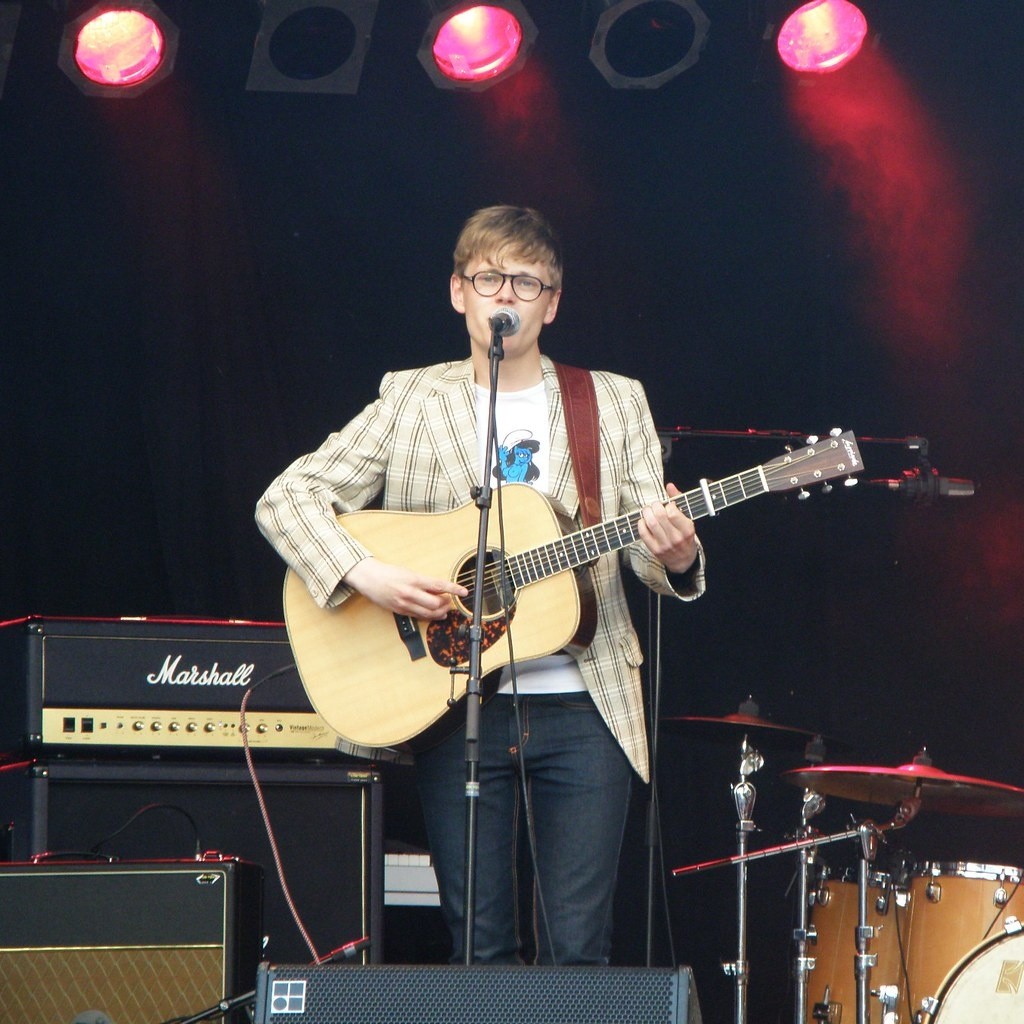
0, 758, 702, 1024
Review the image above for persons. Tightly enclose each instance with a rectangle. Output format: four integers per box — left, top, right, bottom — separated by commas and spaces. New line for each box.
253, 204, 704, 962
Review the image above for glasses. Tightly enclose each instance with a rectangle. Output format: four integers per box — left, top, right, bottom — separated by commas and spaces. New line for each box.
462, 271, 553, 302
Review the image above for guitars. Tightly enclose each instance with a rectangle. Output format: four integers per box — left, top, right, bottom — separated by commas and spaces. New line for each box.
282, 427, 865, 749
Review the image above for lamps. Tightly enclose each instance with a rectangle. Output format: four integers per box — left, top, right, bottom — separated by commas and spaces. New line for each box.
56, 0, 182, 100
403, 0, 541, 97
766, 0, 876, 77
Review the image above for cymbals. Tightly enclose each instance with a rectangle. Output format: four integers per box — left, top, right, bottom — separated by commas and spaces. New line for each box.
661, 695, 821, 741
780, 747, 1024, 819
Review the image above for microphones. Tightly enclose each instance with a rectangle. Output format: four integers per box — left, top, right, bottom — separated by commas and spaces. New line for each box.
71, 1010, 112, 1024
488, 306, 521, 337
873, 476, 975, 496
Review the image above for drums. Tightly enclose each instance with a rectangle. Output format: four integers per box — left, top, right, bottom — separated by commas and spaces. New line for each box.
920, 918, 1023, 1024
805, 864, 909, 1024
899, 861, 1024, 1024
